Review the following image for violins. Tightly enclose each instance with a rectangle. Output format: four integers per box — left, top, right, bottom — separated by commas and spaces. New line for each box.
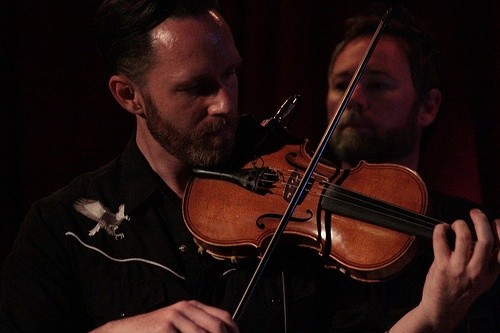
179, 114, 497, 285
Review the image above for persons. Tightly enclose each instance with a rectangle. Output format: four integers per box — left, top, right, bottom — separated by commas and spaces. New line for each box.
0, 0, 500, 333
314, 17, 493, 256
